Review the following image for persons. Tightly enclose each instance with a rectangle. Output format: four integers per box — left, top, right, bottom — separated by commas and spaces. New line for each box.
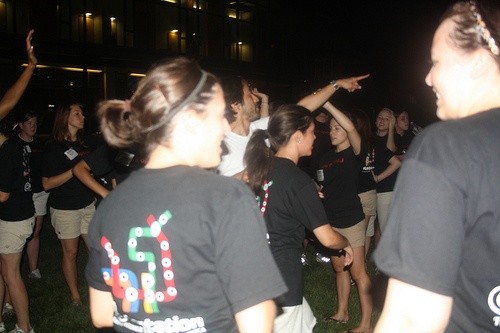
370, 0, 500, 333
0, 30, 423, 333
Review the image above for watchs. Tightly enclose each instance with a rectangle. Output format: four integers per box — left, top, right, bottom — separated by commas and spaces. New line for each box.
329, 80, 339, 91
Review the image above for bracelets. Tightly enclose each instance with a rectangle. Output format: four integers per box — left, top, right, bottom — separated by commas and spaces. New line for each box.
376, 175, 378, 184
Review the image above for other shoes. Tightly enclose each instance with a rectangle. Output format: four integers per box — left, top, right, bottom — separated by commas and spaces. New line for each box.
0, 322, 6, 333
9, 324, 34, 333
29, 269, 42, 278
4, 303, 13, 312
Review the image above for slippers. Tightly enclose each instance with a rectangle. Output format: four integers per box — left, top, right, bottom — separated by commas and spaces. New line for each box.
325, 317, 349, 324
342, 327, 361, 333
